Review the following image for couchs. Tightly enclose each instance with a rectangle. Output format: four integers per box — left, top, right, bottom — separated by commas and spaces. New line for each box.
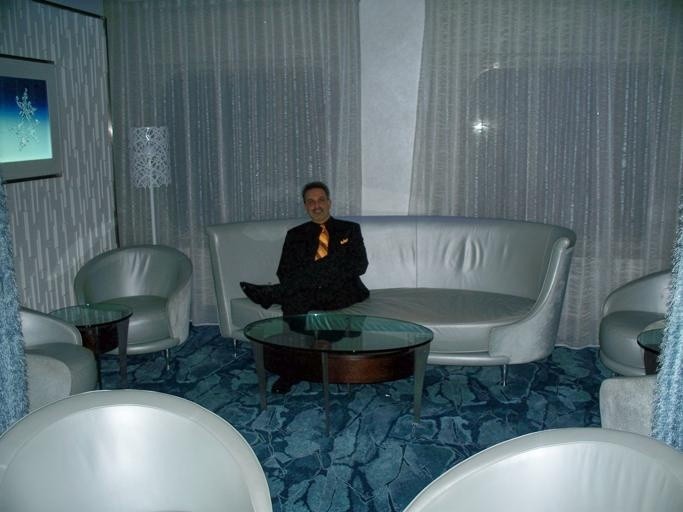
206, 215, 576, 386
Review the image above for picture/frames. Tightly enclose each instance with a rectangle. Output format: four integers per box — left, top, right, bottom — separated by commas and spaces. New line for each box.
0, 54, 64, 186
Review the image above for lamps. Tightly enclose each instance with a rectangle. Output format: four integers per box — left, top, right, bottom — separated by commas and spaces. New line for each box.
128, 126, 170, 245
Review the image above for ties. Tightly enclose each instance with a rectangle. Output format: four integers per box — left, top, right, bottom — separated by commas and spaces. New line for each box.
314, 224, 330, 260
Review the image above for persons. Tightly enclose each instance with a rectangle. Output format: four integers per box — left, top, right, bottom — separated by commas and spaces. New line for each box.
237, 181, 371, 395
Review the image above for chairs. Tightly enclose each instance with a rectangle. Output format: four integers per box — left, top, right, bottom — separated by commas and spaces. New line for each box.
599, 268, 683, 448
402, 422, 683, 512
0, 388, 273, 512
20, 307, 97, 413
74, 245, 193, 370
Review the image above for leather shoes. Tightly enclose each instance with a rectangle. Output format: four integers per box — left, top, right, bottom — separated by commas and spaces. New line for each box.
240, 282, 273, 309
272, 372, 302, 394
